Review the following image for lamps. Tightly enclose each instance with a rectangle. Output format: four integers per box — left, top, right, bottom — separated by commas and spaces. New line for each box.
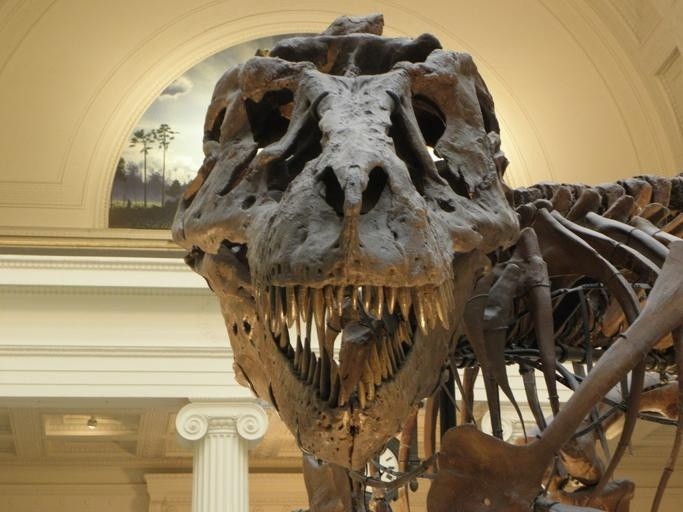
87, 415, 97, 430
